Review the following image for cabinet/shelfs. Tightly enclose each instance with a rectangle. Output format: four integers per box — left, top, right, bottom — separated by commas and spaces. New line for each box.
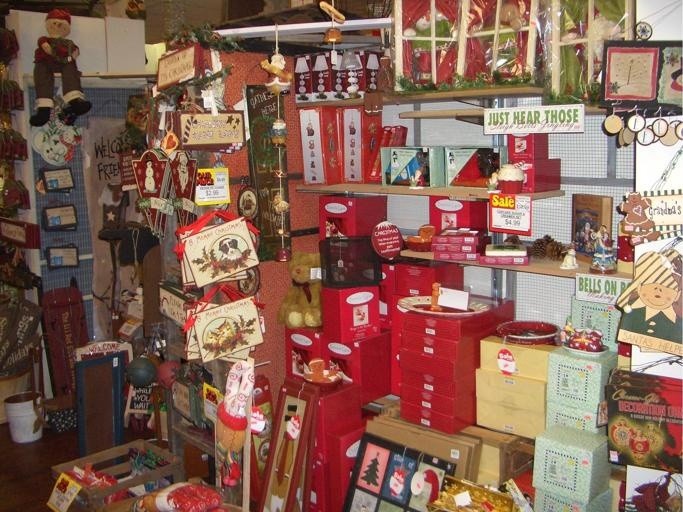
156, 42, 390, 512
291, 0, 682, 512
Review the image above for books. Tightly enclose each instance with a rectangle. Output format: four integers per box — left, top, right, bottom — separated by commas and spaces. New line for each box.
570, 188, 615, 264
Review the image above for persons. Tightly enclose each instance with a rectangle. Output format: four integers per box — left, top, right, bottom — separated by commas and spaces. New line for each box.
28, 9, 91, 127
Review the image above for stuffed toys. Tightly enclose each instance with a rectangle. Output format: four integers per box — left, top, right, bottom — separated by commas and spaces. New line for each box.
277, 250, 325, 330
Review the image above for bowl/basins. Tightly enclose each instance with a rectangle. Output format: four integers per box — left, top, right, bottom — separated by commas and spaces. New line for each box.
494, 320, 562, 345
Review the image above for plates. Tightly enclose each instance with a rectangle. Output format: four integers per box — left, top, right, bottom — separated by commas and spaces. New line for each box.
562, 339, 610, 357
397, 295, 492, 319
